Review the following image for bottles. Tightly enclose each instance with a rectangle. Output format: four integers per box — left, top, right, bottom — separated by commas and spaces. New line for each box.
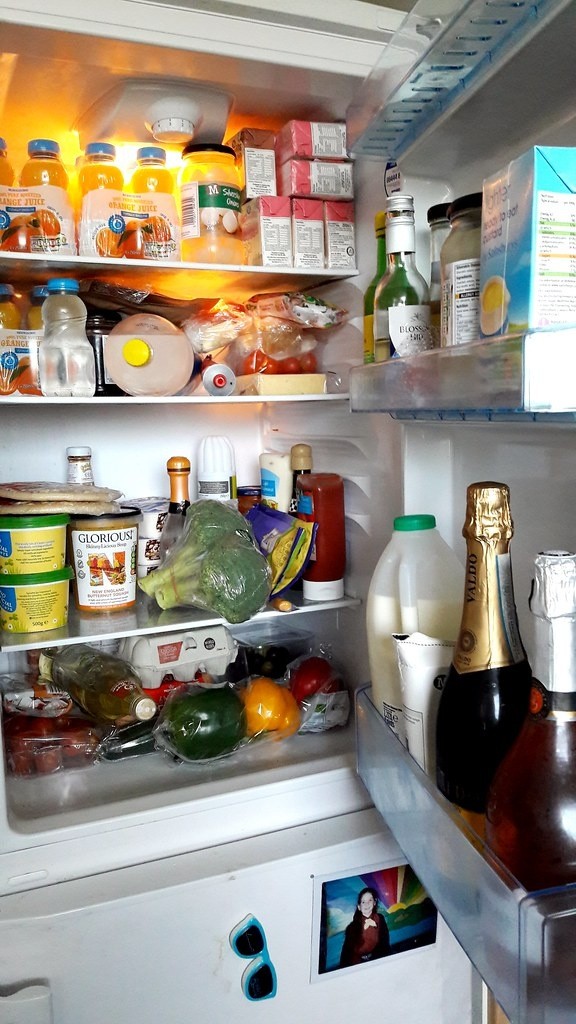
1, 137, 183, 263
365, 512, 466, 755
428, 201, 449, 350
440, 190, 482, 346
63, 447, 101, 590
159, 458, 193, 564
84, 308, 129, 395
288, 443, 312, 521
49, 644, 157, 722
433, 480, 533, 916
373, 195, 435, 364
1, 277, 98, 398
484, 550, 576, 893
105, 313, 194, 398
362, 212, 421, 364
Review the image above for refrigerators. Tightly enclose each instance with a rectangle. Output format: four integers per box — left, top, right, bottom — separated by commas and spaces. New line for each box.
2, 3, 575, 1024
